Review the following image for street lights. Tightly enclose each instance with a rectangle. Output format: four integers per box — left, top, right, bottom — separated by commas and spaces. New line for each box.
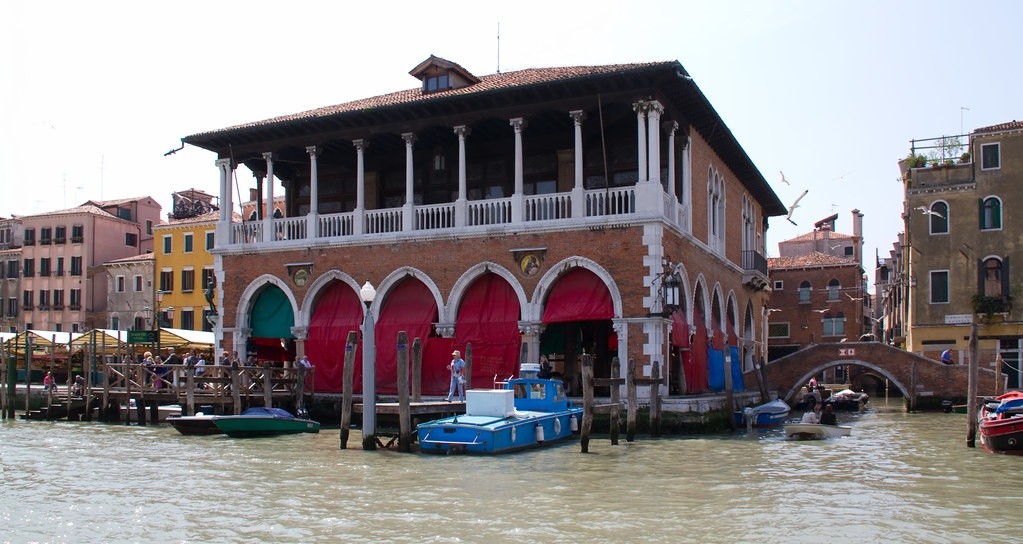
156, 290, 163, 356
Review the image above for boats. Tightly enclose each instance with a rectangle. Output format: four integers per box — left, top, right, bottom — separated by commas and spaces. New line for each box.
735, 400, 791, 429
93, 405, 182, 424
798, 387, 833, 408
212, 407, 320, 437
978, 390, 1023, 452
784, 423, 852, 439
824, 389, 869, 409
165, 411, 222, 436
416, 363, 585, 455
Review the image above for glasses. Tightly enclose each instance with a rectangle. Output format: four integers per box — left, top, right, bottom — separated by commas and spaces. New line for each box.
168, 350, 170, 351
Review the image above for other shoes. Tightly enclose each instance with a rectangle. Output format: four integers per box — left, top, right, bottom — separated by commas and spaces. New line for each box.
445, 399, 451, 403
459, 401, 463, 403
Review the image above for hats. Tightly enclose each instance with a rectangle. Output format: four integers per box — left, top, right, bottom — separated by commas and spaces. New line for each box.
452, 350, 460, 356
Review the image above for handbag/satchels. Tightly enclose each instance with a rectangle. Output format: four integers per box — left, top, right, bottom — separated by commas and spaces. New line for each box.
454, 372, 461, 377
537, 372, 545, 377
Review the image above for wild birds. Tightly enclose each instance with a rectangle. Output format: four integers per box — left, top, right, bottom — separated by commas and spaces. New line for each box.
780, 171, 790, 186
787, 189, 808, 219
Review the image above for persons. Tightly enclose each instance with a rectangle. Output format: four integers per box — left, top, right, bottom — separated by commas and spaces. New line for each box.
539, 355, 550, 379
73, 375, 85, 396
121, 348, 311, 394
205, 276, 216, 313
443, 350, 464, 401
941, 349, 954, 365
801, 377, 837, 426
43, 372, 57, 391
